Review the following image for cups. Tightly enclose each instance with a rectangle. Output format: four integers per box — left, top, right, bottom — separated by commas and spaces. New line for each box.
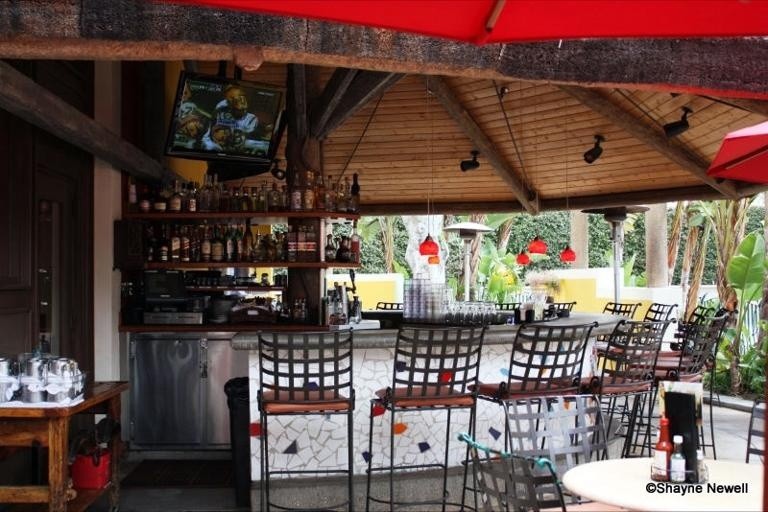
525, 309, 536, 323
402, 269, 442, 325
0, 353, 82, 404
558, 309, 569, 317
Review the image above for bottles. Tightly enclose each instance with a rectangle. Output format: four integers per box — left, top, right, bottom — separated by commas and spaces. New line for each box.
669, 434, 686, 482
652, 418, 673, 482
696, 450, 709, 484
351, 296, 362, 322
153, 219, 362, 263
440, 299, 496, 326
275, 292, 306, 322
139, 171, 360, 212
330, 301, 347, 325
195, 276, 218, 286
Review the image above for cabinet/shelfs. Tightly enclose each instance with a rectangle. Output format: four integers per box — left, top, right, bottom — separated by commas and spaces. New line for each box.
116, 206, 362, 333
0, 380, 131, 511
122, 330, 249, 462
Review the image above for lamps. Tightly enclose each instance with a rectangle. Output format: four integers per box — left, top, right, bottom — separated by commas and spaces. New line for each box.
418, 81, 440, 256
528, 81, 546, 255
427, 78, 440, 265
459, 148, 480, 172
516, 79, 530, 265
659, 103, 693, 137
581, 133, 606, 165
559, 83, 577, 263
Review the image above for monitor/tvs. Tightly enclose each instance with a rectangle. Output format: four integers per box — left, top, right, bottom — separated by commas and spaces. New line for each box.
144, 269, 188, 312
164, 70, 288, 164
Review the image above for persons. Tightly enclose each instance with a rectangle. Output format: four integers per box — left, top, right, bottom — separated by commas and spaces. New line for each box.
173, 81, 271, 158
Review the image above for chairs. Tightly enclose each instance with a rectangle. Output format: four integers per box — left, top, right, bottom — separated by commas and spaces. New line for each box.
254, 299, 764, 511
